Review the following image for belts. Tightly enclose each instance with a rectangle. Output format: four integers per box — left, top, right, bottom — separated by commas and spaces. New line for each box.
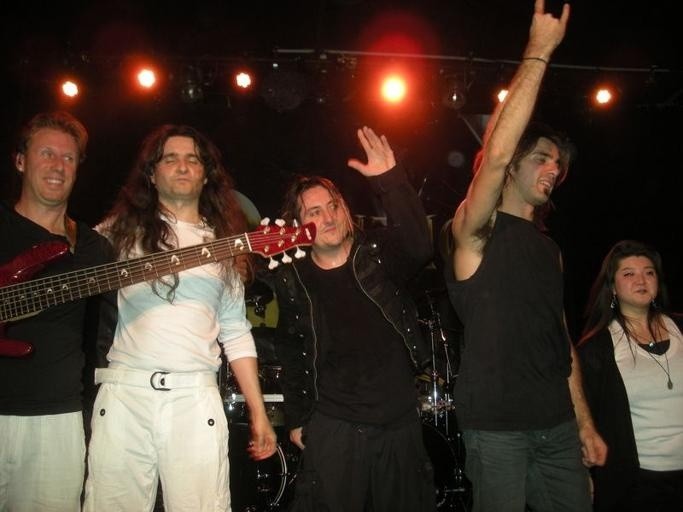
94, 366, 218, 390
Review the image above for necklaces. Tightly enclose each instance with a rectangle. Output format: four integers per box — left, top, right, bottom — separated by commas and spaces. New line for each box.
620, 319, 672, 389
625, 321, 657, 347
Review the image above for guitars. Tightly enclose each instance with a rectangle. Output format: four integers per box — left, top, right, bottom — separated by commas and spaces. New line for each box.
0, 218, 316, 355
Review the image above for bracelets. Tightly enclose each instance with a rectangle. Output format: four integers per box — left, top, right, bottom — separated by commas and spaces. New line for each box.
519, 56, 552, 66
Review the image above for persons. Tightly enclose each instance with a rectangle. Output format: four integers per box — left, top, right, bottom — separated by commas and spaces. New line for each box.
0, 109, 250, 509
77, 120, 280, 512
270, 122, 448, 509
573, 238, 683, 512
446, 0, 609, 509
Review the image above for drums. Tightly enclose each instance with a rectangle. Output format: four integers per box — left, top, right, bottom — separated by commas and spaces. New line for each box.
420, 423, 456, 512
223, 327, 307, 428
227, 422, 301, 512
